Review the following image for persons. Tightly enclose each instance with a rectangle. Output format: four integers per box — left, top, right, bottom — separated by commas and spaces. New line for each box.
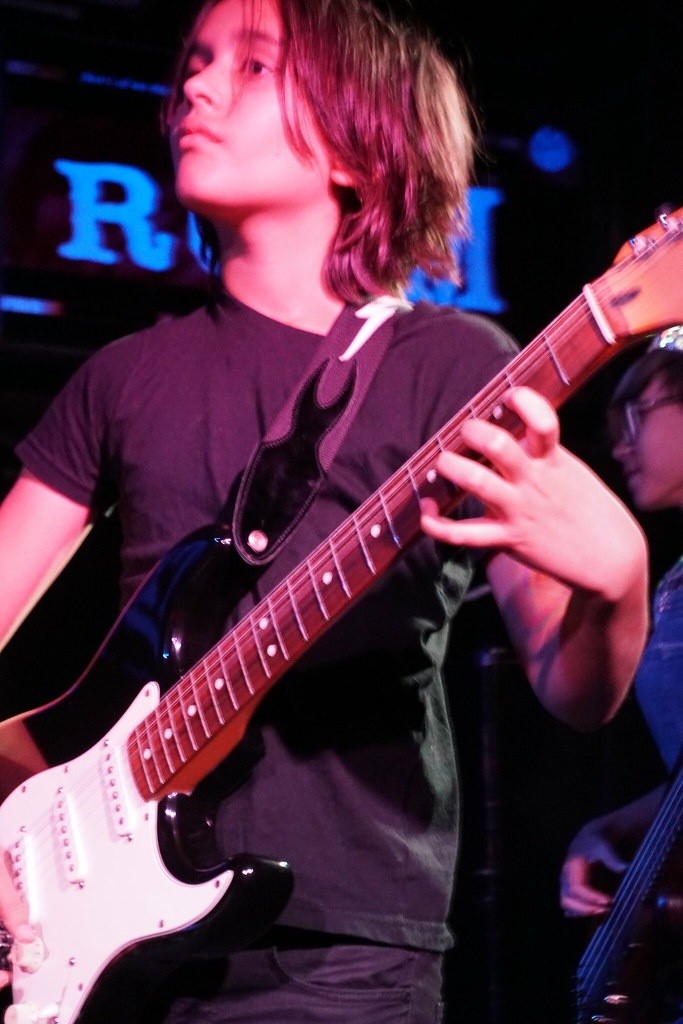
1, 0, 647, 1024
559, 325, 683, 915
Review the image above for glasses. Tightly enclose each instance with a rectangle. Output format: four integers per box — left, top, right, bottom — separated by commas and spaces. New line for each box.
609, 394, 683, 446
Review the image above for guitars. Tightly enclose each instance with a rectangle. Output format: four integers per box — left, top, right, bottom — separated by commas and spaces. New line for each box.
2, 199, 683, 1023
571, 771, 683, 1023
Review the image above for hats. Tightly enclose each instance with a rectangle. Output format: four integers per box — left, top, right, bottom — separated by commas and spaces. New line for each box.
648, 325, 683, 351
